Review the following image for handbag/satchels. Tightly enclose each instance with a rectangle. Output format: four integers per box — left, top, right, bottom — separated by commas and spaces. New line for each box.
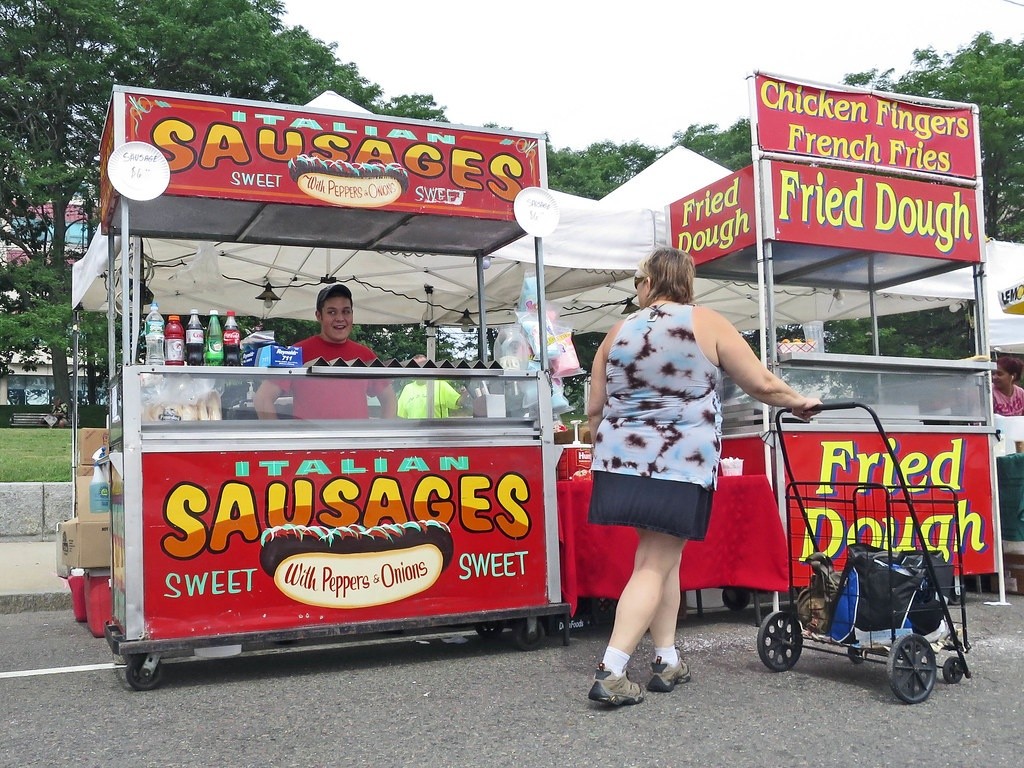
796, 553, 844, 635
826, 543, 956, 647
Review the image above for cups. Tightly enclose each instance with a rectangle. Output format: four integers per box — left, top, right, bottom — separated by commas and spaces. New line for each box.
803, 320, 826, 353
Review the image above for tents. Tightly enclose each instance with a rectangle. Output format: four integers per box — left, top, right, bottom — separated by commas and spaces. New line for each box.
66, 90, 986, 457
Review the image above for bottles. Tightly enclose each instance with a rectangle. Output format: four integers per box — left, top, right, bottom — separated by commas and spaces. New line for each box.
222, 310, 242, 366
145, 301, 166, 365
185, 308, 205, 365
165, 315, 185, 365
205, 310, 225, 366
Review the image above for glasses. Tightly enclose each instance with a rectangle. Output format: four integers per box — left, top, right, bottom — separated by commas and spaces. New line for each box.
635, 276, 645, 289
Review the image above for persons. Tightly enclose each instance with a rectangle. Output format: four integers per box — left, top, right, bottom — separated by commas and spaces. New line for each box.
586, 249, 826, 706
991, 356, 1022, 454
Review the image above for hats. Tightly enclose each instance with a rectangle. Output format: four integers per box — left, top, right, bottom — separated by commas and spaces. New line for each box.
317, 284, 352, 312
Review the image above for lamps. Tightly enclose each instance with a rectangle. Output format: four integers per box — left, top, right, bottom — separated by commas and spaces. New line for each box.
255, 276, 281, 308
456, 307, 476, 333
130, 285, 154, 307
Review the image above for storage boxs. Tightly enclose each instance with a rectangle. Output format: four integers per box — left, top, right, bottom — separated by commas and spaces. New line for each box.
59, 515, 112, 569
76, 475, 112, 524
76, 465, 95, 475
54, 519, 72, 580
79, 427, 110, 463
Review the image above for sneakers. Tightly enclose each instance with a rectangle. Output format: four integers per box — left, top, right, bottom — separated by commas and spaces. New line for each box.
588, 663, 644, 706
646, 647, 691, 693
